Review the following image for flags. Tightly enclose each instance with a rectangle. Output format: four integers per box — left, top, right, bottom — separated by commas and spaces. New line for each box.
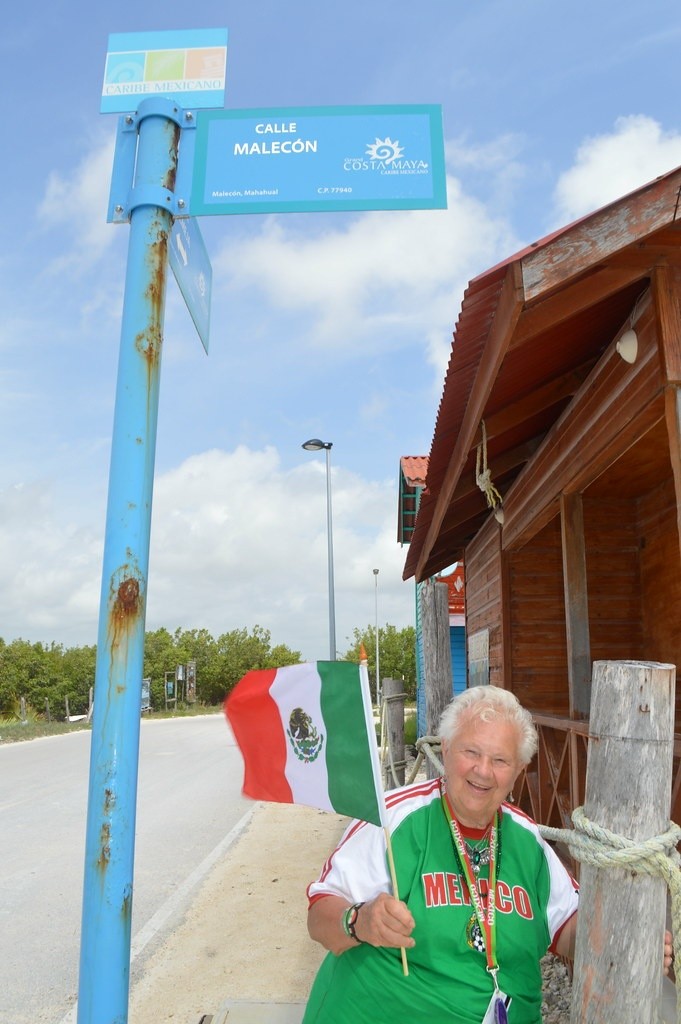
224, 660, 383, 828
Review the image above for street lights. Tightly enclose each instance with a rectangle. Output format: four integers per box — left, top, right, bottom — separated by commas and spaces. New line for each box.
372, 568, 381, 709
301, 438, 338, 661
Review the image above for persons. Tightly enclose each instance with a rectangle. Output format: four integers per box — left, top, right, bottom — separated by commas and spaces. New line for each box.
299, 684, 675, 1023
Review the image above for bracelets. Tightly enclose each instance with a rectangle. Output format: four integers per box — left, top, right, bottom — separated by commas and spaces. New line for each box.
343, 902, 368, 943
